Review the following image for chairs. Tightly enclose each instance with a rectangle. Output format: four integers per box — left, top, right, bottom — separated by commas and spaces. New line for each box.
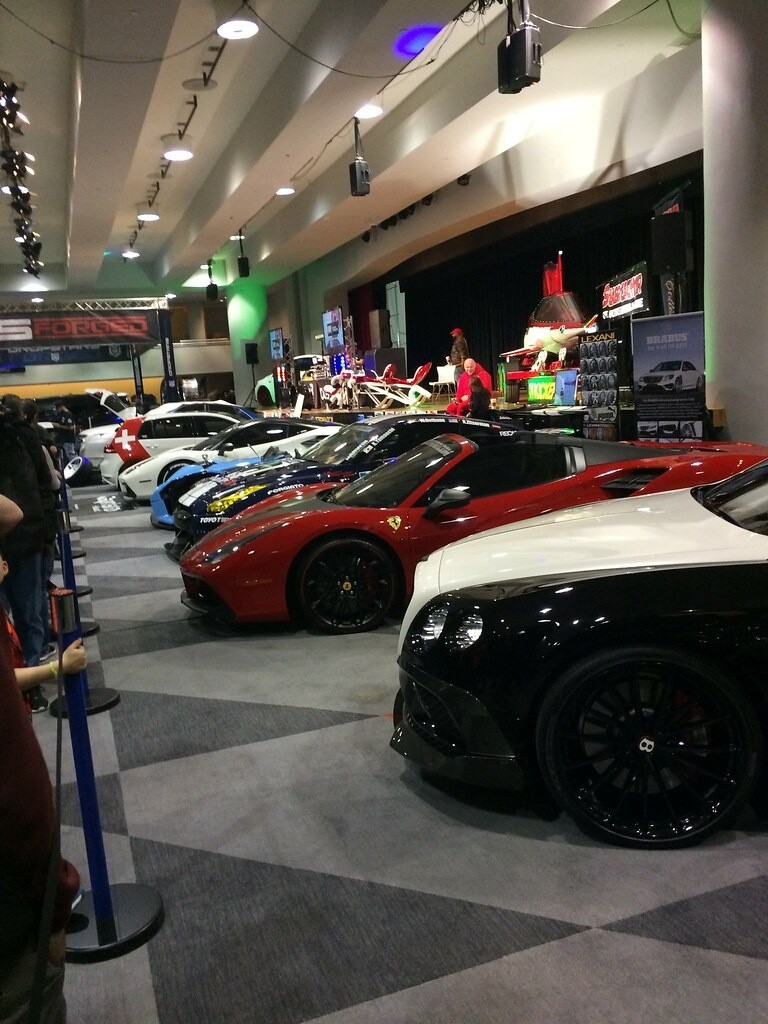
429, 366, 456, 404
322, 363, 396, 410
386, 361, 432, 408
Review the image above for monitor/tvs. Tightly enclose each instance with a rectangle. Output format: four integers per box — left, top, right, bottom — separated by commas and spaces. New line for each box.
553, 369, 579, 406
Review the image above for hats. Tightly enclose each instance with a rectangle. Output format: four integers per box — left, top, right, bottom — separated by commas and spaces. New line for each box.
450, 328, 462, 335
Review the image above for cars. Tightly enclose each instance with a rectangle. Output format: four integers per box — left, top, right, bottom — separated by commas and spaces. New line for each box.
117, 416, 347, 504
84, 388, 239, 485
255, 355, 330, 406
638, 360, 702, 393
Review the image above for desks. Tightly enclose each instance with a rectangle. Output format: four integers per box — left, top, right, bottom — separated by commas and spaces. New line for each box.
297, 378, 331, 410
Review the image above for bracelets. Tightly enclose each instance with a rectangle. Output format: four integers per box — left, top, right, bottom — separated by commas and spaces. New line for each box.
49, 662, 57, 681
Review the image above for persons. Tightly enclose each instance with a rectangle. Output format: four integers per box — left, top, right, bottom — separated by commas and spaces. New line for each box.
345, 341, 364, 407
0, 394, 87, 1024
446, 358, 491, 417
466, 376, 491, 419
327, 321, 340, 347
53, 401, 79, 462
450, 328, 469, 384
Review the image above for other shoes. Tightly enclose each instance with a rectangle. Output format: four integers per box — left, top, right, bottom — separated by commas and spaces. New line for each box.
39, 645, 56, 661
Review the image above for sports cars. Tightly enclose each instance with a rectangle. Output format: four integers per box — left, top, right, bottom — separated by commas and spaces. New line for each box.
150, 411, 530, 562
392, 455, 768, 850
178, 431, 768, 635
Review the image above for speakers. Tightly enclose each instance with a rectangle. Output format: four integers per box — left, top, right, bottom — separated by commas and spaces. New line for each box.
237, 257, 249, 277
496, 28, 542, 94
206, 284, 218, 301
652, 211, 695, 276
349, 161, 370, 196
245, 343, 258, 364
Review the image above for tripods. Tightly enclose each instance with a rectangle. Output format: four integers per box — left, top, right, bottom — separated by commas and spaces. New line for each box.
243, 363, 256, 407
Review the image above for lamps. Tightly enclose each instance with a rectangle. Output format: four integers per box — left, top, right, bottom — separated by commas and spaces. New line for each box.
362, 231, 370, 242
275, 181, 295, 196
457, 173, 471, 186
238, 228, 251, 278
206, 259, 219, 300
497, 0, 543, 96
422, 194, 433, 206
354, 93, 383, 119
161, 134, 193, 161
0, 81, 43, 279
349, 116, 371, 198
380, 214, 398, 230
136, 201, 160, 222
399, 204, 416, 219
121, 242, 141, 259
215, 3, 260, 40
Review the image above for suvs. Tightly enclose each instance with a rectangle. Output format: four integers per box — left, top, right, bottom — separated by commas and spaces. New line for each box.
77, 399, 256, 471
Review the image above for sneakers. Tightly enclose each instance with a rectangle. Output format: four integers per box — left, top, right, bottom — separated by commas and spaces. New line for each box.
29, 687, 48, 712
